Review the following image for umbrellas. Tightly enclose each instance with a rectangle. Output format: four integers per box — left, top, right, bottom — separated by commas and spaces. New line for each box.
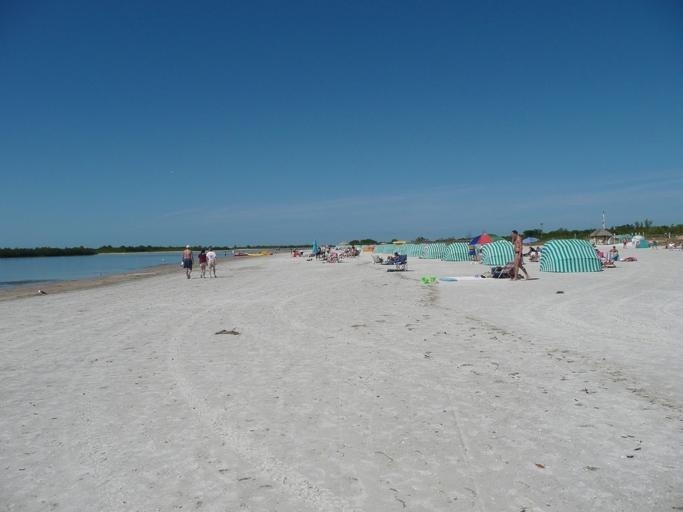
313, 240, 318, 261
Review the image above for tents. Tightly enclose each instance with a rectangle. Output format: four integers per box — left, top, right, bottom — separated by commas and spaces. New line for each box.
540, 238, 602, 272
636, 239, 648, 248
363, 232, 515, 266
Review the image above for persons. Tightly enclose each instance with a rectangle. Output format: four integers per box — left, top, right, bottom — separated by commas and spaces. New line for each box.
205, 248, 217, 278
649, 234, 683, 249
512, 230, 529, 281
288, 245, 357, 264
521, 246, 542, 262
181, 245, 193, 279
590, 237, 640, 270
198, 249, 207, 279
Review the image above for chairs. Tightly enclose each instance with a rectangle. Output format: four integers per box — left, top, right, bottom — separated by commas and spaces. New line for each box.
493, 262, 516, 279
